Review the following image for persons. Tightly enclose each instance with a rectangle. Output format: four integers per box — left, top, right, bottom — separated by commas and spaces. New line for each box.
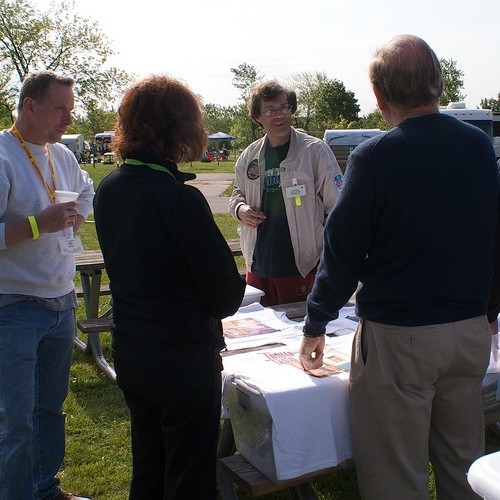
92, 76, 246, 500
225, 79, 345, 307
221, 148, 228, 161
299, 34, 500, 500
0, 73, 95, 500
83, 140, 97, 162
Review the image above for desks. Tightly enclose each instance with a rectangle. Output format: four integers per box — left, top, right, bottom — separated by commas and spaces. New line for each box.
75, 238, 243, 353
220, 301, 500, 483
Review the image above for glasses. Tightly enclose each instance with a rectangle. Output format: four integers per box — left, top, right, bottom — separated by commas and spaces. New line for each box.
259, 104, 293, 118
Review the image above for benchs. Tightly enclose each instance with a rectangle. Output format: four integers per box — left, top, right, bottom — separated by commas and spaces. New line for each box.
75, 284, 117, 383
220, 409, 500, 497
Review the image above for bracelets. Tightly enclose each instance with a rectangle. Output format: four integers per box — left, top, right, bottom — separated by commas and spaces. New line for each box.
27, 216, 40, 240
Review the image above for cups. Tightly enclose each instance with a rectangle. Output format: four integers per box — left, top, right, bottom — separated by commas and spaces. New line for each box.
55, 191, 80, 206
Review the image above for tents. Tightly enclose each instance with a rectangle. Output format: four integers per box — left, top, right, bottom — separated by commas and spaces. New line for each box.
207, 132, 236, 165
